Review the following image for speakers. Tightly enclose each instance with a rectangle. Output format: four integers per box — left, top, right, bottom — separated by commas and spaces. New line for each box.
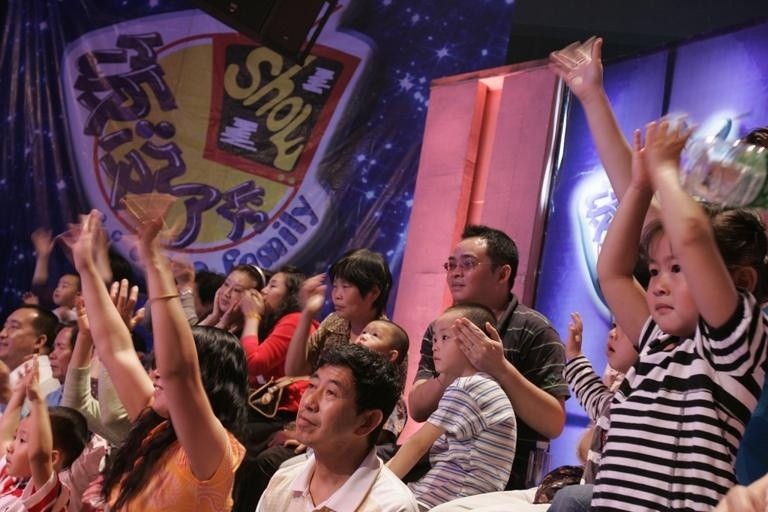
190, 0, 336, 66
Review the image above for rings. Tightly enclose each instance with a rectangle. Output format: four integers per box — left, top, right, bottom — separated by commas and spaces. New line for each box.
467, 344, 472, 350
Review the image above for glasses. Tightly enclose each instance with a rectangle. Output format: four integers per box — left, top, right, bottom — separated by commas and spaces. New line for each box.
444, 260, 501, 272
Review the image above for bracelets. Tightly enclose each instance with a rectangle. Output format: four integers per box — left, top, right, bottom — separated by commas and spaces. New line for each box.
436, 376, 446, 389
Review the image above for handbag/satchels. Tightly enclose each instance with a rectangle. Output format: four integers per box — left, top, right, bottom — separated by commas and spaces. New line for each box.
247, 375, 311, 418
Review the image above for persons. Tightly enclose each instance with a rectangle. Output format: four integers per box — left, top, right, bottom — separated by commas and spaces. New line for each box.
526, 29, 768, 511
376, 222, 571, 494
385, 305, 517, 512
1, 200, 420, 512
